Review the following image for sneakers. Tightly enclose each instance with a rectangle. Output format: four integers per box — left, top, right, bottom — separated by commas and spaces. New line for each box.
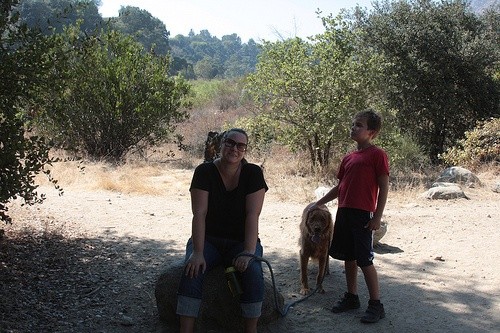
332, 291, 360, 313
360, 300, 385, 323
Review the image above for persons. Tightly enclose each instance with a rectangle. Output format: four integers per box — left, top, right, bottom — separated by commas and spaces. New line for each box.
307, 107, 390, 325
176, 128, 268, 333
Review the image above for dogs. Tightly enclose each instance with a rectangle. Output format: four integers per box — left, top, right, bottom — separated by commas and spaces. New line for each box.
298, 201, 334, 297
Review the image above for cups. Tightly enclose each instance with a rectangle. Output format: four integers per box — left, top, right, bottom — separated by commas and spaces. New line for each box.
225, 267, 243, 297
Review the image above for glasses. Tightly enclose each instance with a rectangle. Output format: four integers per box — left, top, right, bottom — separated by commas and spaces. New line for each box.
224, 137, 247, 152
